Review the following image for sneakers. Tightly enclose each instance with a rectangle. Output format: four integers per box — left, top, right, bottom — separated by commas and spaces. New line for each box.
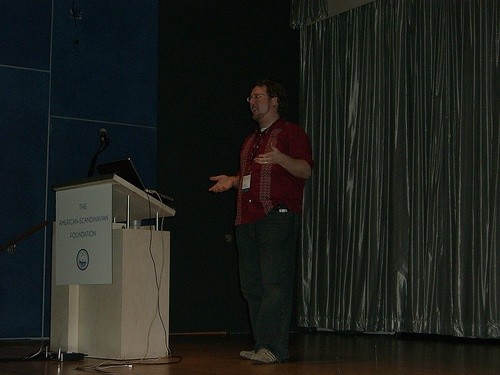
251, 344, 281, 363
240, 350, 287, 361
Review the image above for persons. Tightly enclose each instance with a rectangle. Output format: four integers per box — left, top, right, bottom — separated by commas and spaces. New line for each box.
209, 81, 314, 364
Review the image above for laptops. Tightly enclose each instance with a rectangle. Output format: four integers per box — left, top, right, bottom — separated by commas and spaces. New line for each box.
98, 158, 175, 202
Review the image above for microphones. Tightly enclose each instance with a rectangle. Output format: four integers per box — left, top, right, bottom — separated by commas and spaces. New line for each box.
98, 128, 108, 142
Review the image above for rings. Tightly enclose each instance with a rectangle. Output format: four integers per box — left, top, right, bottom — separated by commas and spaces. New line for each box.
265, 161, 267, 165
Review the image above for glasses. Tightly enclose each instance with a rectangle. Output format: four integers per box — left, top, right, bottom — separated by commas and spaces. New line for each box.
247, 94, 276, 102
